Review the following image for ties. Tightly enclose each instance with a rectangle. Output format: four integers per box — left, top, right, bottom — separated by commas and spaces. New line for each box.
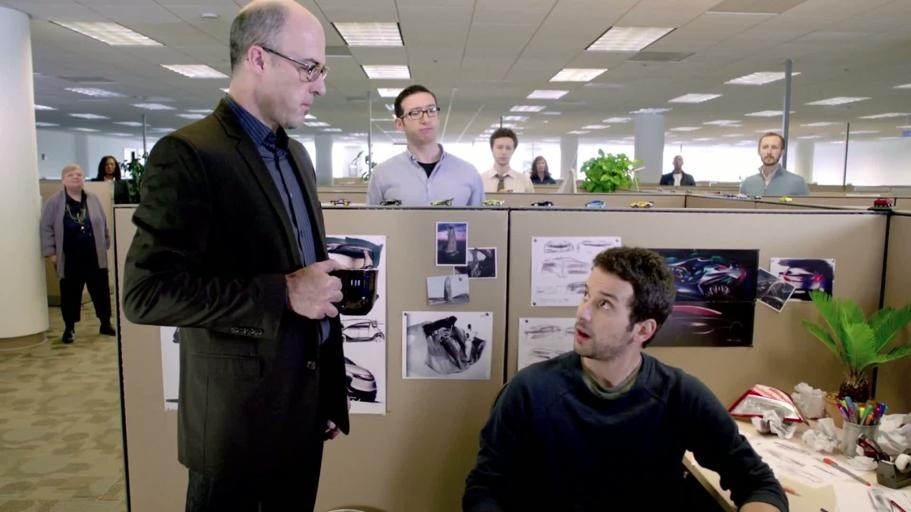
262, 141, 334, 347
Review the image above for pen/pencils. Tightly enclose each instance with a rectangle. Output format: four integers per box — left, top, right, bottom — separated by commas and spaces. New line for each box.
781, 485, 803, 498
886, 498, 906, 512
823, 457, 871, 487
836, 395, 889, 426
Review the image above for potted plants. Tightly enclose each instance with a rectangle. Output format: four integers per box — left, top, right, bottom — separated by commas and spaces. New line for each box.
802, 288, 909, 430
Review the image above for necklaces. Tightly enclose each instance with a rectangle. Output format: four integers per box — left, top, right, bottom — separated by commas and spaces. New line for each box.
66, 203, 88, 225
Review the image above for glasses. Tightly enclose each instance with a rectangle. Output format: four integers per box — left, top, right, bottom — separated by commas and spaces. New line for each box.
258, 42, 330, 83
399, 106, 442, 123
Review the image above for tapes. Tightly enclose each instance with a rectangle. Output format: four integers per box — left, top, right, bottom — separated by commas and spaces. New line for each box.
895, 453, 911, 471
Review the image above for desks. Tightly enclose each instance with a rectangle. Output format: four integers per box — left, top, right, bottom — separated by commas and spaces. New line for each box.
679, 410, 908, 512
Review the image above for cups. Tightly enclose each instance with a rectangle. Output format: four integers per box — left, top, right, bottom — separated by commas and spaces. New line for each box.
328, 269, 378, 316
843, 420, 882, 459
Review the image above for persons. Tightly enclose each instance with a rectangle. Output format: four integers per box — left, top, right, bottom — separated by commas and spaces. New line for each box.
530, 156, 557, 185
91, 156, 129, 204
739, 132, 809, 197
464, 323, 477, 362
368, 84, 485, 206
122, 1, 351, 511
479, 127, 535, 193
40, 164, 115, 344
462, 247, 789, 512
660, 155, 696, 187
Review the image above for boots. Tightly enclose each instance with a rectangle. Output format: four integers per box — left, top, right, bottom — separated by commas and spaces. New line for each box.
62, 320, 77, 343
97, 312, 118, 337
